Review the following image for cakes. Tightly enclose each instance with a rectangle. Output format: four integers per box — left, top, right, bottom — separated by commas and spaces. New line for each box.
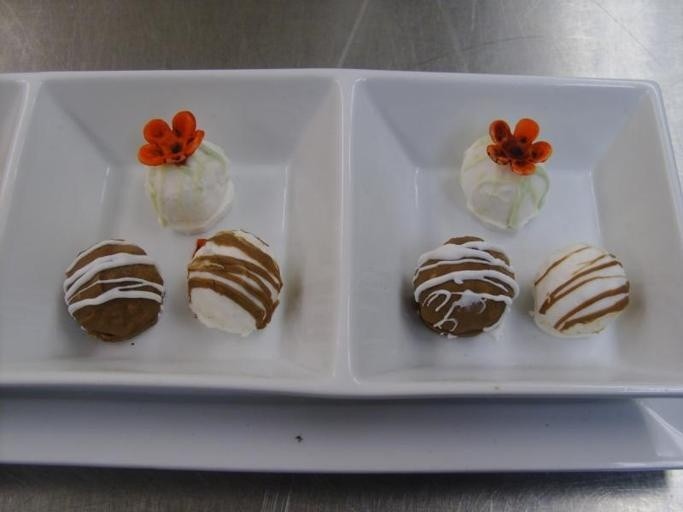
414, 236, 518, 338
528, 245, 630, 336
61, 238, 165, 340
186, 228, 284, 335
139, 111, 235, 234
460, 118, 552, 229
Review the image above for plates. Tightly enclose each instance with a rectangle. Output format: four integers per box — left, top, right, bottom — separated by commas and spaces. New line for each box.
0, 68, 683, 403
